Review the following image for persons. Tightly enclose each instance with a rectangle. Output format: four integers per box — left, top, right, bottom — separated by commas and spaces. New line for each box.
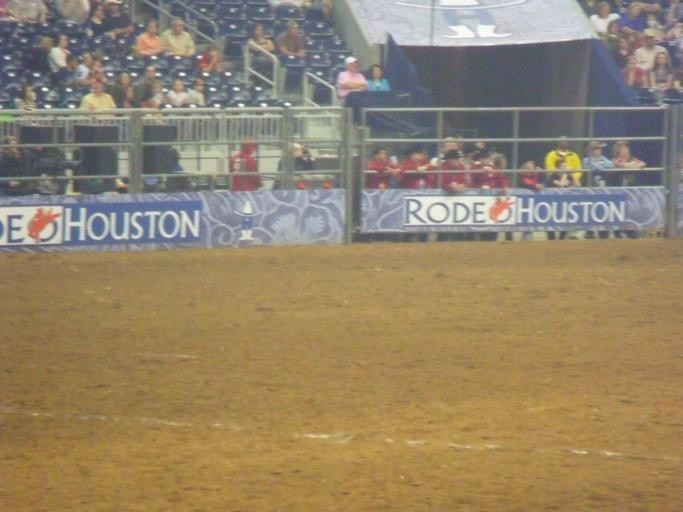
550, 160, 580, 187
367, 64, 390, 92
520, 158, 545, 191
580, 0, 682, 107
542, 135, 582, 179
1, 0, 332, 195
336, 56, 368, 102
611, 140, 646, 187
366, 135, 513, 194
581, 138, 613, 187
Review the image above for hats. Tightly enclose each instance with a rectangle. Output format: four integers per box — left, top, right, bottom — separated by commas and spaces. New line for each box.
345, 56, 359, 65
641, 29, 657, 38
591, 141, 607, 149
